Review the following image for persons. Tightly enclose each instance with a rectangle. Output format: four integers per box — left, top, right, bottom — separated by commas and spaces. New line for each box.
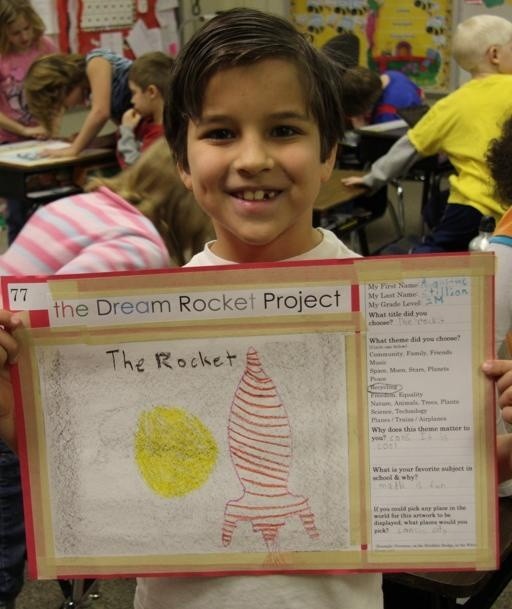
23, 47, 132, 159
0, 6, 510, 606
341, 65, 426, 163
0, 131, 217, 608
341, 14, 510, 253
116, 50, 174, 170
467, 119, 512, 609
1, 1, 62, 247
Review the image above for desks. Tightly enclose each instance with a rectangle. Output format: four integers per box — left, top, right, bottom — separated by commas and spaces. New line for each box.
0, 140, 115, 250
312, 118, 453, 258
